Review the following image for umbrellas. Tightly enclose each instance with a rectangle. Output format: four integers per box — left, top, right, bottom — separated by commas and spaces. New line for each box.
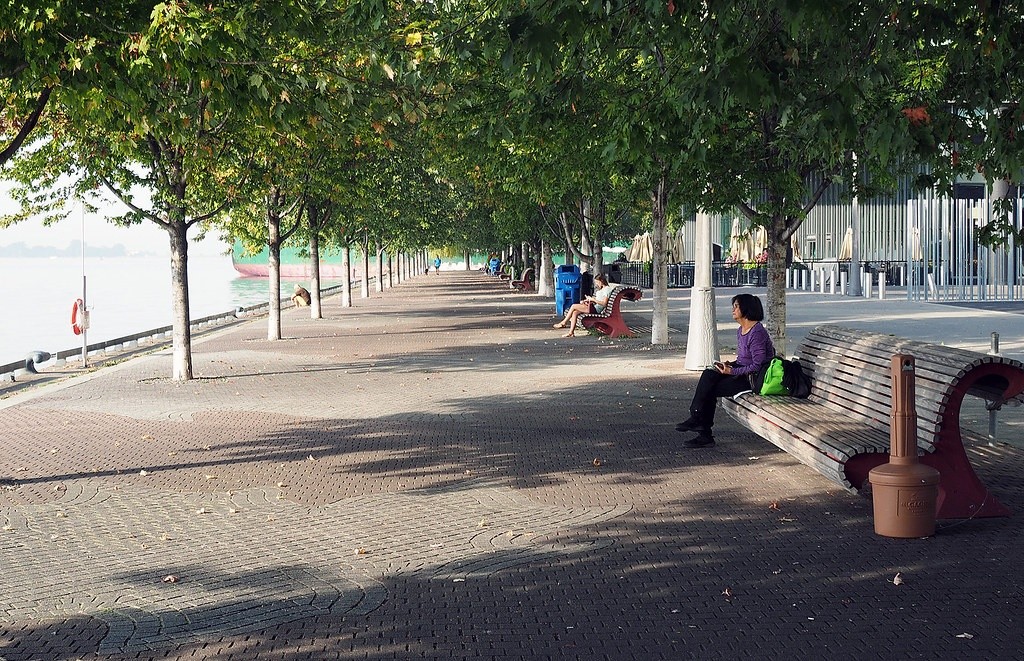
911, 225, 923, 285
730, 219, 798, 263
630, 228, 686, 264
839, 228, 853, 282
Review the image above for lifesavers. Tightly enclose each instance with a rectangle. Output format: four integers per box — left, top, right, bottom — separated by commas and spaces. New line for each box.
71, 298, 83, 336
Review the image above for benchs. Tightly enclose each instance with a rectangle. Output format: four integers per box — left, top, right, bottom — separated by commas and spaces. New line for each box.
493, 264, 534, 291
720, 325, 1024, 520
564, 283, 645, 338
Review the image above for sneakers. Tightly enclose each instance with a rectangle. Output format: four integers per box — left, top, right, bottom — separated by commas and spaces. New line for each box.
676, 416, 706, 432
683, 433, 716, 447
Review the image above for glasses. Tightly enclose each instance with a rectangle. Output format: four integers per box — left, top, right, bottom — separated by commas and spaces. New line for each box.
732, 304, 740, 308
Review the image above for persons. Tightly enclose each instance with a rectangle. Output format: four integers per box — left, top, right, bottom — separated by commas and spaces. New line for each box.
755, 250, 768, 262
552, 261, 555, 269
553, 274, 612, 338
485, 250, 500, 276
434, 255, 441, 275
291, 284, 311, 309
674, 293, 775, 447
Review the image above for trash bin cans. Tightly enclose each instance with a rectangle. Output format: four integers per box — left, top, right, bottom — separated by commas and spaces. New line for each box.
554, 265, 581, 316
490, 259, 500, 275
602, 264, 622, 284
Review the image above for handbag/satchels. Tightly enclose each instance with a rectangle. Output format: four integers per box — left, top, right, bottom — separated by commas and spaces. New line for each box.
760, 358, 791, 395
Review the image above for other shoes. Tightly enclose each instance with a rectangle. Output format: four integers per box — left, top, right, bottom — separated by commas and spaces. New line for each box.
553, 322, 567, 328
562, 333, 575, 338
